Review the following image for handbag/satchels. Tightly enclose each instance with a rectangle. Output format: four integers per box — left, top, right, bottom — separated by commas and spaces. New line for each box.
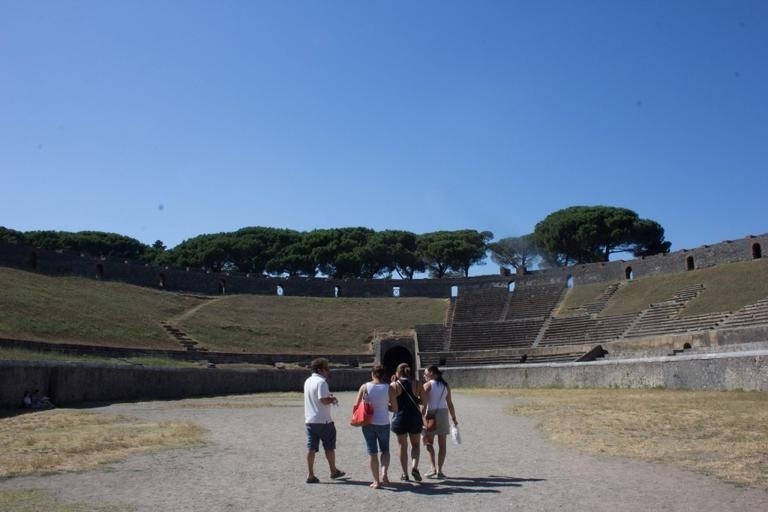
452, 423, 461, 445
350, 384, 374, 427
424, 414, 436, 433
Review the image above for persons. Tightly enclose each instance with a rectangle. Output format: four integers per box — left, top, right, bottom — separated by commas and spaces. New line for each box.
353, 363, 459, 489
23, 389, 55, 410
303, 358, 346, 483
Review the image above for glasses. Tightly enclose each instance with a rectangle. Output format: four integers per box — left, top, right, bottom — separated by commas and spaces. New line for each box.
323, 367, 328, 371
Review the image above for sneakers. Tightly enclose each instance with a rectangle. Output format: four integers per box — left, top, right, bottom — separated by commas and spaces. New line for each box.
382, 475, 390, 487
412, 467, 422, 482
369, 483, 381, 489
424, 472, 448, 479
305, 476, 320, 483
400, 473, 409, 482
330, 469, 346, 479
